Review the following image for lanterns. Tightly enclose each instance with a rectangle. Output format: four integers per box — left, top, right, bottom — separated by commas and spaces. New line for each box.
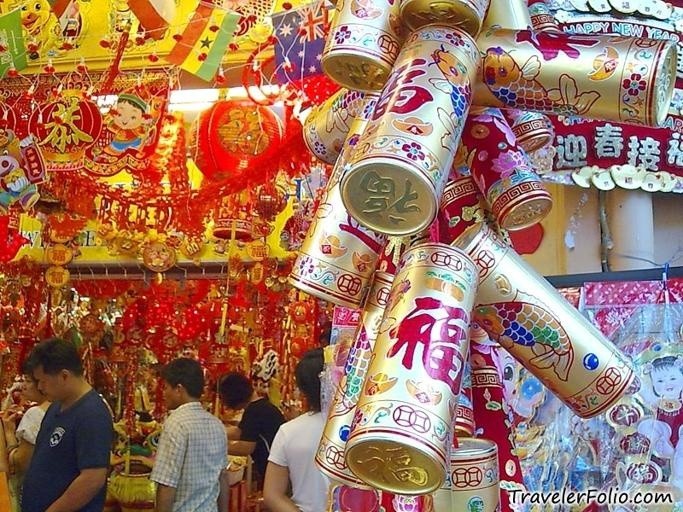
252, 183, 287, 221
27, 90, 103, 172
189, 95, 285, 184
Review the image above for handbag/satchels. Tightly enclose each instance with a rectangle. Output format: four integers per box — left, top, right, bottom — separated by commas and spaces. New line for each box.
7, 474, 22, 512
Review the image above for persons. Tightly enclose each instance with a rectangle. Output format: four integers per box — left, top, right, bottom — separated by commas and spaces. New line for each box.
21, 338, 114, 512
150, 357, 228, 512
215, 372, 286, 477
1, 363, 52, 512
262, 347, 330, 512
